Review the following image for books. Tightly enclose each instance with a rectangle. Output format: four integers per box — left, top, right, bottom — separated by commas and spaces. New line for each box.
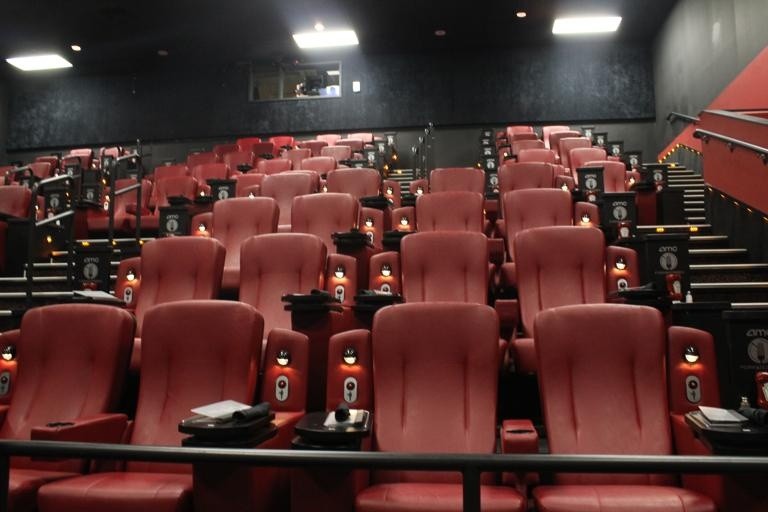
696, 405, 749, 432
74, 290, 120, 298
190, 400, 252, 425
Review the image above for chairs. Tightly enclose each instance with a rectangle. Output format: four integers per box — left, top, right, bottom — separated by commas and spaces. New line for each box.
70, 148, 93, 169
353, 231, 489, 305
503, 148, 554, 165
99, 144, 124, 170
499, 139, 546, 166
384, 192, 487, 250
347, 133, 374, 150
278, 145, 311, 170
0, 184, 31, 275
287, 193, 386, 262
328, 166, 401, 229
41, 297, 311, 512
559, 136, 591, 175
243, 172, 314, 232
375, 135, 385, 140
233, 174, 267, 198
238, 137, 259, 151
85, 178, 152, 229
66, 154, 88, 174
195, 164, 230, 197
270, 136, 295, 157
1, 176, 7, 185
36, 155, 58, 178
497, 163, 576, 234
335, 138, 366, 159
153, 165, 190, 206
1, 304, 135, 494
535, 303, 720, 511
214, 142, 239, 162
299, 140, 328, 156
195, 197, 280, 288
496, 133, 538, 151
225, 152, 254, 173
301, 156, 337, 192
286, 301, 526, 512
550, 131, 580, 162
254, 158, 292, 173
322, 146, 353, 169
570, 147, 620, 186
542, 125, 570, 149
74, 236, 226, 370
497, 126, 533, 139
116, 177, 196, 229
514, 226, 639, 372
27, 162, 52, 220
318, 135, 340, 146
503, 189, 600, 288
254, 142, 274, 160
411, 167, 486, 194
186, 152, 219, 174
236, 233, 358, 372
582, 161, 640, 196
0, 160, 22, 175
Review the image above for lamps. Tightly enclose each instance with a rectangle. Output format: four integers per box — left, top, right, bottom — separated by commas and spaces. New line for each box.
292, 29, 355, 48
70, 44, 81, 52
315, 23, 323, 30
3, 55, 74, 72
551, 1, 619, 44
516, 10, 527, 19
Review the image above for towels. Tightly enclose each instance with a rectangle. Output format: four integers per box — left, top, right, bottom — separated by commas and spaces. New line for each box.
334, 398, 349, 423
310, 287, 330, 295
738, 405, 768, 423
230, 400, 272, 425
